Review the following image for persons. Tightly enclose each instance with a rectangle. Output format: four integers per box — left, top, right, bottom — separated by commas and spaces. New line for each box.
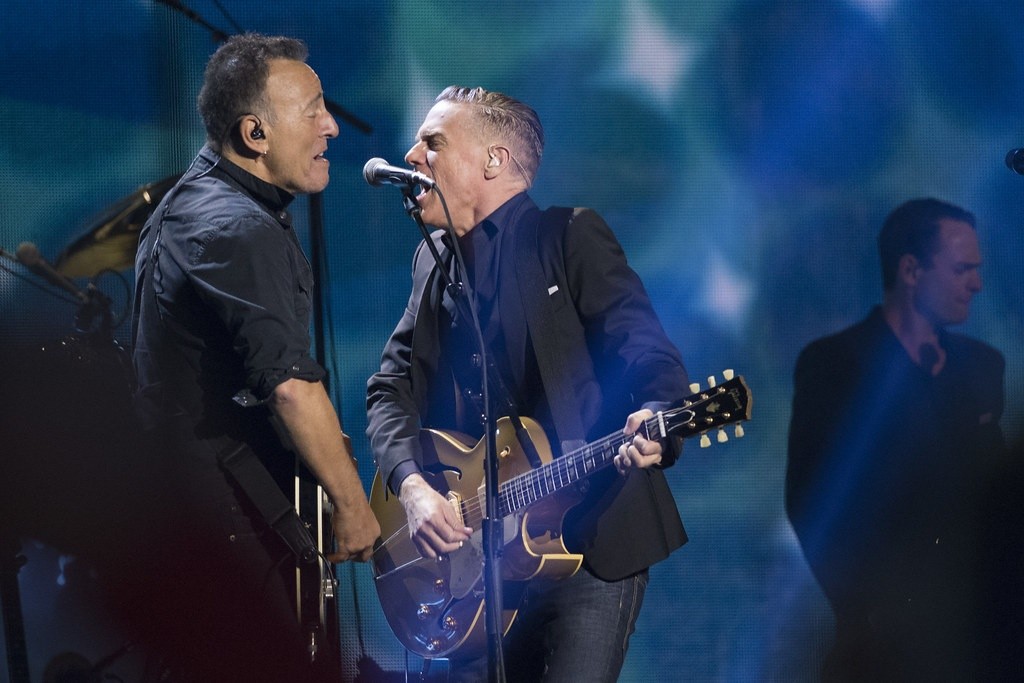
366, 85, 692, 683
132, 33, 382, 683
784, 198, 1024, 683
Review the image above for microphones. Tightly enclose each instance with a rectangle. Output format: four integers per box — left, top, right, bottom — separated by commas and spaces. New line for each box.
363, 156, 437, 189
1004, 148, 1024, 176
15, 242, 89, 304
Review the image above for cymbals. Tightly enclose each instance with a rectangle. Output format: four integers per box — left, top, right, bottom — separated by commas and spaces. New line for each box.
55, 169, 185, 280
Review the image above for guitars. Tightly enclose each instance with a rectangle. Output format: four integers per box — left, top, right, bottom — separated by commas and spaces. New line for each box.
284, 411, 351, 665
353, 362, 755, 668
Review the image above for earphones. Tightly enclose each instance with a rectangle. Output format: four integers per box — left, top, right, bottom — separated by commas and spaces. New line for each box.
251, 128, 263, 140
488, 153, 501, 168
